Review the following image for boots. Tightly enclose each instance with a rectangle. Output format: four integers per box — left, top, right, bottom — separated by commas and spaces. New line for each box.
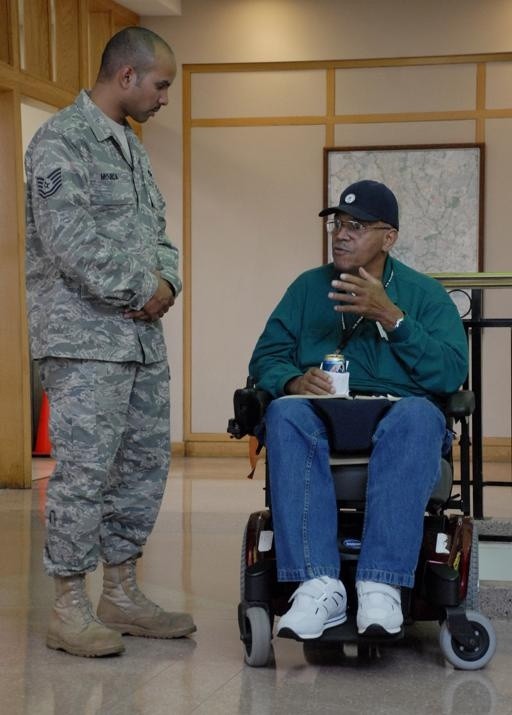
45, 576, 125, 658
97, 558, 197, 638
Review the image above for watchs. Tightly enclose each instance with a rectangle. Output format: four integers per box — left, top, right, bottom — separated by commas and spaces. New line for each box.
393, 309, 406, 330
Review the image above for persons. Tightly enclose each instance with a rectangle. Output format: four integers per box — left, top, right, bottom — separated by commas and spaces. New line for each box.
249, 179, 472, 643
22, 25, 199, 660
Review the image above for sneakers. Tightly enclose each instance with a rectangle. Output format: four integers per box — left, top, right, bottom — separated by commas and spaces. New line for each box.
355, 581, 404, 636
275, 576, 347, 642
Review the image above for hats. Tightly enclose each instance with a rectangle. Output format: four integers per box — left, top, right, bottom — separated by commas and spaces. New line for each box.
319, 180, 399, 230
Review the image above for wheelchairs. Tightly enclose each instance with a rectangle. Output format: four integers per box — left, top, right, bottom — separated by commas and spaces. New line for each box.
228, 370, 498, 671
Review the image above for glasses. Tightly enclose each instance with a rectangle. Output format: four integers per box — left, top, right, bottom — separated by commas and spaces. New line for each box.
324, 218, 391, 234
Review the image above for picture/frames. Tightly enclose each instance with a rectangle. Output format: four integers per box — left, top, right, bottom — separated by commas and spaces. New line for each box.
324, 142, 485, 322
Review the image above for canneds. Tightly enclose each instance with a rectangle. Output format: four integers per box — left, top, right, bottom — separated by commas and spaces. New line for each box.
323, 353, 346, 375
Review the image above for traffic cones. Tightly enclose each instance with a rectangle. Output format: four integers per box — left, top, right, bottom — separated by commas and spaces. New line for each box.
33, 455, 58, 528
32, 391, 52, 457
